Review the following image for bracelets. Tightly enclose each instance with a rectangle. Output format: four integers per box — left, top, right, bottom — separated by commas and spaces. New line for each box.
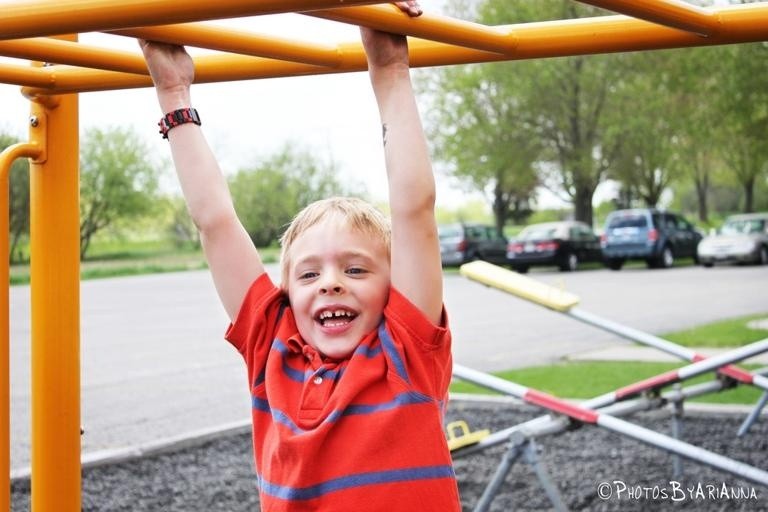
158, 108, 201, 139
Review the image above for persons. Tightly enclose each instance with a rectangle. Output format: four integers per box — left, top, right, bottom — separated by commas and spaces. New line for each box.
136, 2, 463, 512
